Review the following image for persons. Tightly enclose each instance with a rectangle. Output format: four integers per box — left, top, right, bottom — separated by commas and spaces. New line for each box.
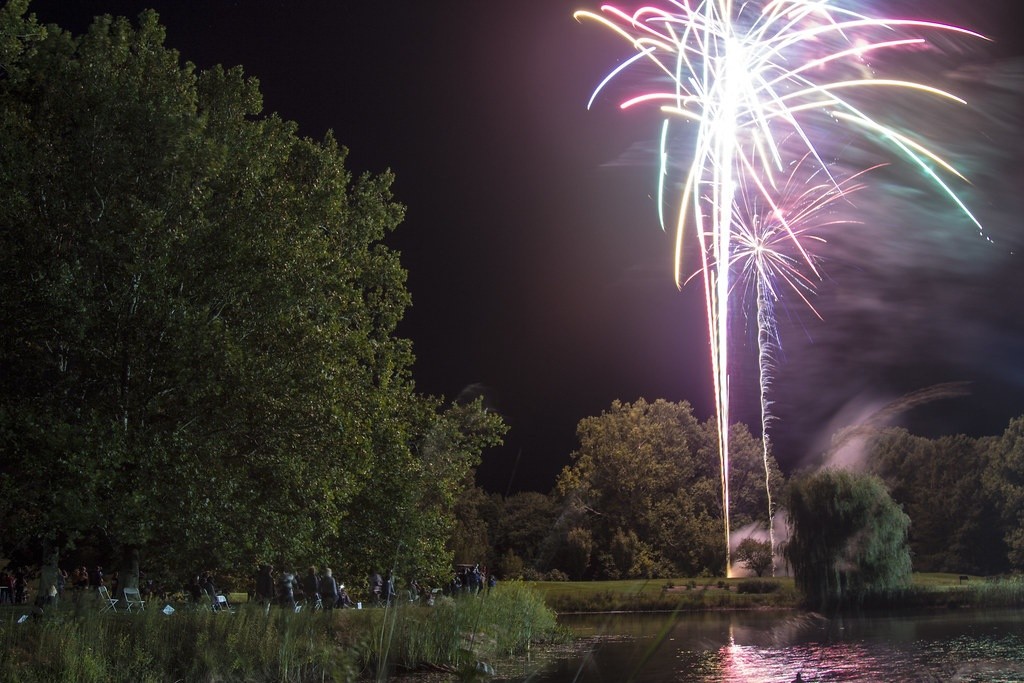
0, 564, 497, 610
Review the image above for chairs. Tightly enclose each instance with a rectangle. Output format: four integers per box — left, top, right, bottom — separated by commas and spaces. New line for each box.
204, 584, 232, 612
99, 586, 119, 613
124, 587, 145, 614
